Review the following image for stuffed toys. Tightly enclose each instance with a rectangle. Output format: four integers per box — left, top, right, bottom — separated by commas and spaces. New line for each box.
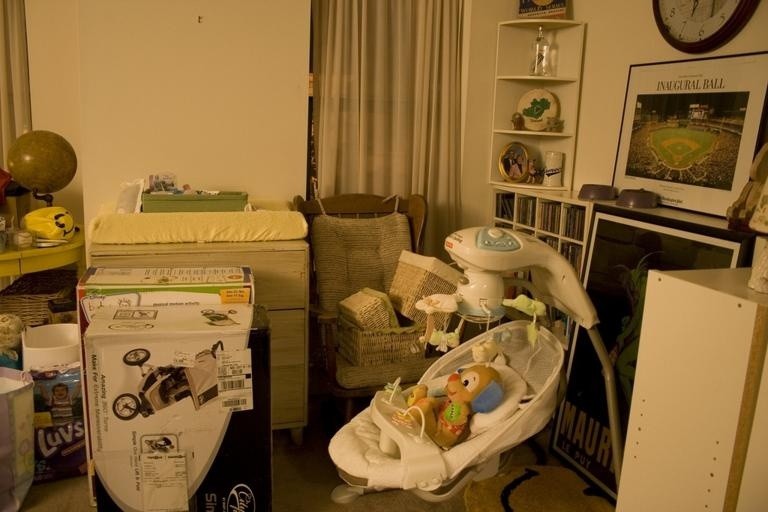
407, 363, 504, 448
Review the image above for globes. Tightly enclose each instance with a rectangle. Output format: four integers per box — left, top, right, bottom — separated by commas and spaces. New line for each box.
7, 130, 81, 232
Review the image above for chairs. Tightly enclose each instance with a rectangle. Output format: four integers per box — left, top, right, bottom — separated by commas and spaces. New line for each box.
292, 193, 430, 431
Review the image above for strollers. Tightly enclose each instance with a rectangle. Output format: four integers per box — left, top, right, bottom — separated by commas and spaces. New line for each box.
112, 340, 225, 421
200, 308, 241, 330
145, 436, 175, 453
325, 221, 627, 512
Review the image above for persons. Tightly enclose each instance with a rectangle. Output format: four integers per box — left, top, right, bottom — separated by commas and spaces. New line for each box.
39, 382, 82, 432
504, 150, 517, 172
509, 155, 526, 178
614, 233, 676, 409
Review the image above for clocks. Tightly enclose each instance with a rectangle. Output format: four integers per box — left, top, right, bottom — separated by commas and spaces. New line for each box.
650, 1, 764, 54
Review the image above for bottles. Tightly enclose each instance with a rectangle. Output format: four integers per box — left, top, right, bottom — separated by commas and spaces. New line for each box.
529, 25, 550, 77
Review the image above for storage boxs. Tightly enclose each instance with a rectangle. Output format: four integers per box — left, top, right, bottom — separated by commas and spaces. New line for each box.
81, 304, 273, 512
74, 267, 255, 506
141, 190, 247, 213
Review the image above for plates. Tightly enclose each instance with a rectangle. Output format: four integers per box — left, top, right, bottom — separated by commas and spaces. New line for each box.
517, 88, 558, 131
34, 243, 59, 248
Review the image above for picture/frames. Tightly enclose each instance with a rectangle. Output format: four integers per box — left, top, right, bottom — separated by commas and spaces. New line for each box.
551, 202, 752, 507
606, 50, 768, 222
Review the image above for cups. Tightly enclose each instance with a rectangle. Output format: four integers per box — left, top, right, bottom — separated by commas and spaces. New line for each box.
543, 151, 563, 187
746, 235, 768, 293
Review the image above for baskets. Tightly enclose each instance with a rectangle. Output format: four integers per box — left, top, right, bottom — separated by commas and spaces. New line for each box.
338, 251, 465, 366
0, 274, 77, 325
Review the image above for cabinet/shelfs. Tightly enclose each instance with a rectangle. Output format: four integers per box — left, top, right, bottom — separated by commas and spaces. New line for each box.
485, 19, 586, 191
611, 265, 768, 510
89, 241, 309, 449
488, 186, 590, 356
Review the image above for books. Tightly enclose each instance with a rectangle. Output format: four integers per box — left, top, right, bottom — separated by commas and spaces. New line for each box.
496, 194, 586, 342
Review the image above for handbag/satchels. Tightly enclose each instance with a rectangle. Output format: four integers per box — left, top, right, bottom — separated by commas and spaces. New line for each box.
0, 367, 35, 512
